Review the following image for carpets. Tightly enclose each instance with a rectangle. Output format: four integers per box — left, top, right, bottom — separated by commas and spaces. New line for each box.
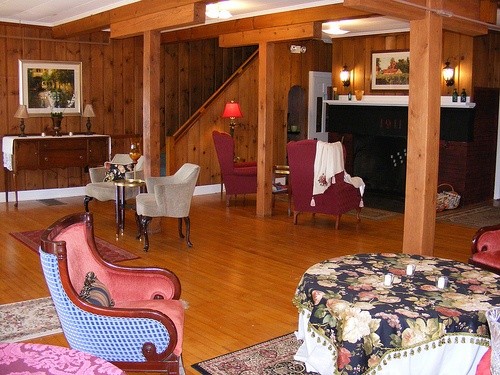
190, 330, 320, 375
9, 229, 142, 263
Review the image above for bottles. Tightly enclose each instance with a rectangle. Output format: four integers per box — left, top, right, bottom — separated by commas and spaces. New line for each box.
461, 89, 466, 102
348, 93, 352, 100
453, 89, 457, 102
106, 171, 114, 182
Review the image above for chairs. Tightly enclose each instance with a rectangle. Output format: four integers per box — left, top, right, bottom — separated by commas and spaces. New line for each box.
468, 224, 500, 276
286, 139, 365, 230
89, 167, 106, 183
134, 163, 201, 252
212, 130, 257, 206
39, 212, 185, 375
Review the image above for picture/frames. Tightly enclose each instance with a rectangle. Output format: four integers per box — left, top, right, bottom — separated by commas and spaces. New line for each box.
370, 49, 410, 92
340, 64, 350, 87
17, 59, 83, 118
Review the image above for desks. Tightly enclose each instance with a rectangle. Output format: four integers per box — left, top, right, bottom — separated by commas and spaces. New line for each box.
292, 252, 500, 375
2, 132, 112, 207
0, 343, 126, 375
273, 166, 291, 217
83, 179, 147, 231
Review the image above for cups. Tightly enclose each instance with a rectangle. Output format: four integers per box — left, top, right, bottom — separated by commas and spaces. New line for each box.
327, 86, 337, 100
381, 273, 394, 287
404, 264, 416, 276
290, 126, 297, 132
125, 172, 134, 181
435, 274, 449, 288
485, 307, 500, 375
355, 91, 364, 100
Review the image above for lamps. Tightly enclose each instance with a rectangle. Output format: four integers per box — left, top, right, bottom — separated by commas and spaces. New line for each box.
441, 62, 455, 87
221, 99, 242, 139
14, 105, 29, 137
82, 104, 96, 134
290, 44, 306, 54
